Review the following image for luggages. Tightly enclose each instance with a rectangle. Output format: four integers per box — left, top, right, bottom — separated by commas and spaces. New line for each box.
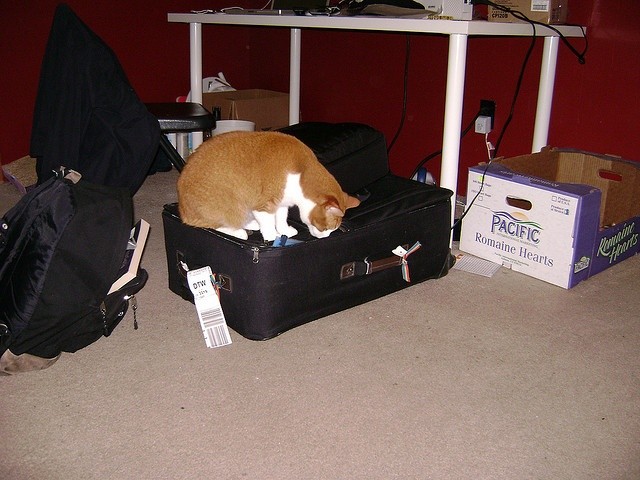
162, 121, 454, 341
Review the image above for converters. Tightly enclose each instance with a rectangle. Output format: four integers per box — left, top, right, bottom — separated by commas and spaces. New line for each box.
475, 116, 491, 134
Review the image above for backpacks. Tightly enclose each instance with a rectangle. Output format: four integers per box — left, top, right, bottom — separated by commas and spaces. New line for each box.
0, 166, 148, 359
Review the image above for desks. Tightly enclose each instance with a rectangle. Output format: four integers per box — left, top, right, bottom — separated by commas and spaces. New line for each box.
166, 12, 588, 252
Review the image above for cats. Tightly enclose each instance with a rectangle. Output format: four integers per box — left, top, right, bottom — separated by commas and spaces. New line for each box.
176, 130, 361, 240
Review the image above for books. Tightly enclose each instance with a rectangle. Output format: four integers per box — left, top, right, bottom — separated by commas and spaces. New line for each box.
106, 218, 150, 295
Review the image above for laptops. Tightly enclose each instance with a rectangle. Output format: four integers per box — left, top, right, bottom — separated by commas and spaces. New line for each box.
224, 0, 330, 15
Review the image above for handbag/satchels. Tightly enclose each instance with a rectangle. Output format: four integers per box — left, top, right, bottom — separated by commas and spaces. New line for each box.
274, 120, 389, 203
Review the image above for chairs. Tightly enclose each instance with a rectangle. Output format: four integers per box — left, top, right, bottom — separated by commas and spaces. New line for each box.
145, 102, 217, 175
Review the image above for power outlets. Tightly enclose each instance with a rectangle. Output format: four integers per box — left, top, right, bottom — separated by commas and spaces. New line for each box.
479, 99, 497, 130
